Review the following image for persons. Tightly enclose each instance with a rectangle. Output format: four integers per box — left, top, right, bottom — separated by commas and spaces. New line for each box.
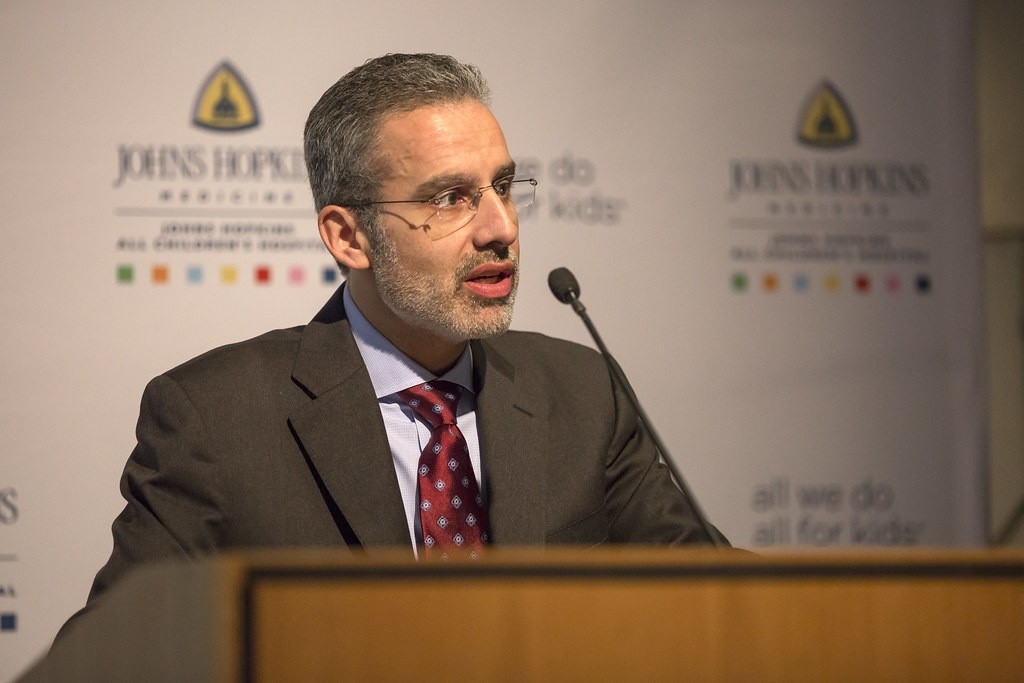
48, 51, 731, 656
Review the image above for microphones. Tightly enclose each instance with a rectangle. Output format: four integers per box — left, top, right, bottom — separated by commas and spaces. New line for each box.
549, 267, 723, 547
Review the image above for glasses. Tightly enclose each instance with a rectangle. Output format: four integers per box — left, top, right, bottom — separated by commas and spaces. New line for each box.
336, 176, 538, 208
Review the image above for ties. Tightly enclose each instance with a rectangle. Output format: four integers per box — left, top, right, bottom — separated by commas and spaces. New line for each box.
396, 380, 492, 560
